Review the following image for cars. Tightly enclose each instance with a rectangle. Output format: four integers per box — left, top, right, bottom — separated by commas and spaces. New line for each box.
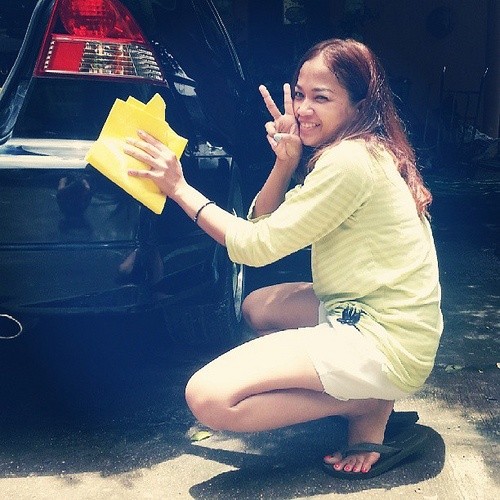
0, 0, 250, 368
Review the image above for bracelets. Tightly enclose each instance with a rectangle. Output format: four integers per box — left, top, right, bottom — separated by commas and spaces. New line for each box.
192, 200, 215, 224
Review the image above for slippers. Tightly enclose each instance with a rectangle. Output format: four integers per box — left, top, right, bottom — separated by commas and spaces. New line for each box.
322, 411, 429, 480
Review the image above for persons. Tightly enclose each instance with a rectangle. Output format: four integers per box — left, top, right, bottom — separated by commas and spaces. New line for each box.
123, 38, 445, 474
